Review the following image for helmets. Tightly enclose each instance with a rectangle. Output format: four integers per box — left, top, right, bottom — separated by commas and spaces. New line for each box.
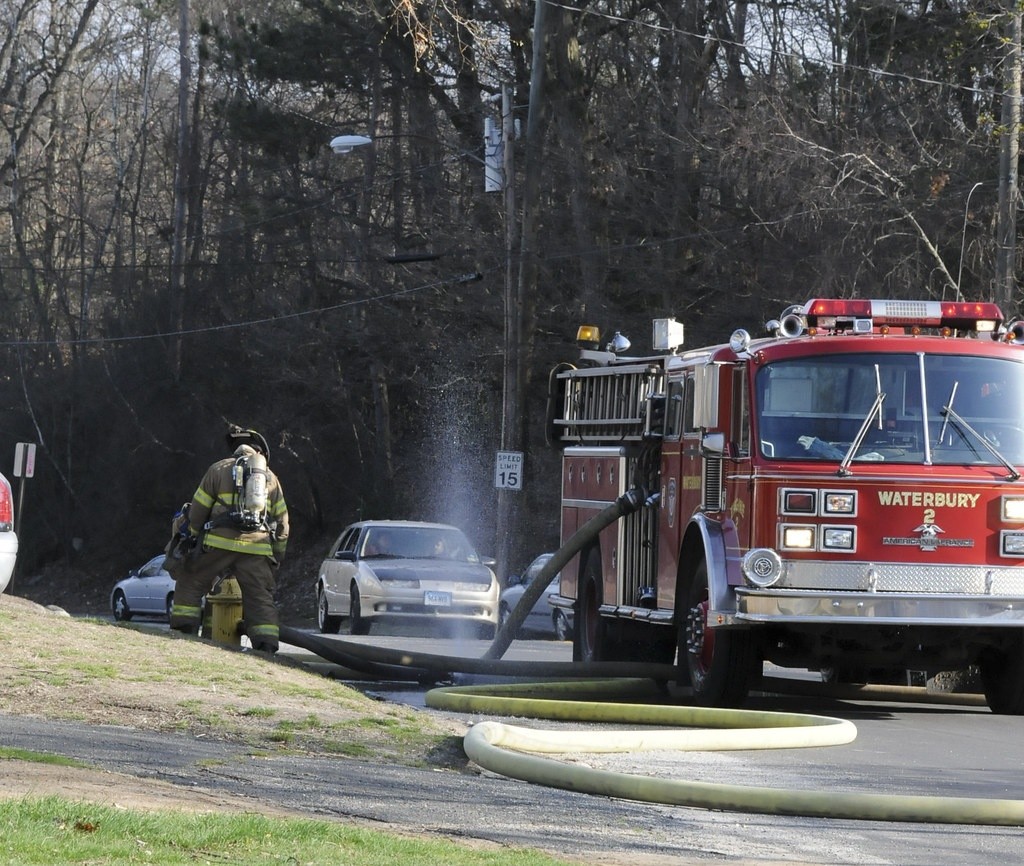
226, 429, 270, 465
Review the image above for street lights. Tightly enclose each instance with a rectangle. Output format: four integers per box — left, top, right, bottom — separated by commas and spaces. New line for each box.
329, 132, 521, 598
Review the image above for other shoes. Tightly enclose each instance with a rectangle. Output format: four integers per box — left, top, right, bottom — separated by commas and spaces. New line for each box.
260, 645, 274, 656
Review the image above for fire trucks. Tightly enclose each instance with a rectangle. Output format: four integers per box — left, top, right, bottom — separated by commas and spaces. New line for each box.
544, 293, 1024, 718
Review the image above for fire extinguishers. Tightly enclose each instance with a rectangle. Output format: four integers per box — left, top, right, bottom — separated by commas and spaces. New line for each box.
235, 452, 268, 526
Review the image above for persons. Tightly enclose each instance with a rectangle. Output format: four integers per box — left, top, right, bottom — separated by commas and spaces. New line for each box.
365, 529, 400, 555
418, 535, 453, 560
162, 429, 289, 656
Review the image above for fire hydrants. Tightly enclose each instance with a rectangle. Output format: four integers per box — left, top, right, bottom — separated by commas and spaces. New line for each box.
206, 573, 248, 649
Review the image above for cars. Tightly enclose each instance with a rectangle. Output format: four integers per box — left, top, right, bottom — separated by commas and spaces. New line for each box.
110, 553, 206, 625
497, 551, 578, 643
315, 517, 499, 641
